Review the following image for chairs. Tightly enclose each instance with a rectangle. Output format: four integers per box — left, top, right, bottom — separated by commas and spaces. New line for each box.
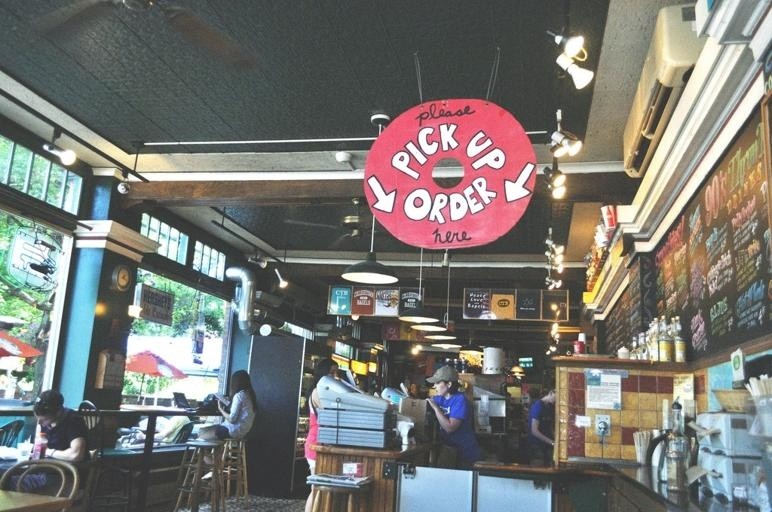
2, 458, 80, 512
1, 419, 29, 446
160, 421, 198, 446
78, 400, 133, 512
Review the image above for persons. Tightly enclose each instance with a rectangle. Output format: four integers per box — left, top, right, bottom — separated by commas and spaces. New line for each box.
528, 378, 556, 465
426, 365, 485, 469
197, 370, 258, 479
304, 360, 340, 512
16, 389, 91, 491
117, 398, 191, 446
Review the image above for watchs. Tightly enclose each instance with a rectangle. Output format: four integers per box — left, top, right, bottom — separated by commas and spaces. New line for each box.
47, 448, 56, 457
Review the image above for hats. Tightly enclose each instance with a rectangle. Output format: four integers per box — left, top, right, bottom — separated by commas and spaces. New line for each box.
425, 364, 459, 384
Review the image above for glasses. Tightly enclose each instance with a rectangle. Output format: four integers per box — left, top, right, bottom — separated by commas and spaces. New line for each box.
33, 397, 52, 406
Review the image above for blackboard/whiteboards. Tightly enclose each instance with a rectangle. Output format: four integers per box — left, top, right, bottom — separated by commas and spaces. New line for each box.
593, 95, 771, 371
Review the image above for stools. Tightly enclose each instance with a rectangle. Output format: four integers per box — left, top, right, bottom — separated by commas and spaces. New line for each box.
173, 439, 225, 512
221, 437, 254, 504
309, 484, 370, 512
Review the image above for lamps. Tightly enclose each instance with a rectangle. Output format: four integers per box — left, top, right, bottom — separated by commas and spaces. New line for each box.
509, 366, 526, 373
545, 277, 562, 289
544, 280, 553, 290
247, 246, 267, 270
545, 238, 563, 255
428, 341, 463, 350
410, 278, 448, 333
551, 262, 564, 273
460, 339, 483, 354
544, 322, 561, 355
544, 30, 588, 62
275, 267, 289, 289
423, 333, 456, 341
398, 249, 442, 324
551, 140, 565, 158
340, 114, 400, 285
552, 130, 583, 158
43, 127, 79, 168
545, 251, 564, 264
542, 166, 567, 186
555, 52, 594, 90
546, 183, 566, 200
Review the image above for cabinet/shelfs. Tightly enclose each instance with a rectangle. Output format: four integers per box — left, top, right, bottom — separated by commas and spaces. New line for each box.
310, 431, 444, 512
553, 355, 695, 465
396, 460, 611, 512
608, 463, 762, 511
247, 335, 384, 502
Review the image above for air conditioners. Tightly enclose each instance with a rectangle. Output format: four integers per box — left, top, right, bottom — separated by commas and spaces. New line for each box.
622, 5, 709, 179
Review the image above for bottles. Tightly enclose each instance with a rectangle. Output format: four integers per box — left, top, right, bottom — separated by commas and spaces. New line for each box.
657, 314, 671, 367
666, 403, 689, 459
629, 335, 640, 361
32, 432, 47, 467
673, 319, 686, 364
637, 318, 668, 360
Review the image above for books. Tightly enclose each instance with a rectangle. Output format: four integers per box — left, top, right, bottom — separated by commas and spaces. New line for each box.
306, 473, 372, 488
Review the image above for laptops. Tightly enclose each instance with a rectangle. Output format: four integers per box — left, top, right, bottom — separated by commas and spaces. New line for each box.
174, 392, 196, 411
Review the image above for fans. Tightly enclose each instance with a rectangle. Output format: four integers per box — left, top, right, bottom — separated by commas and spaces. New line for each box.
28, 0, 246, 71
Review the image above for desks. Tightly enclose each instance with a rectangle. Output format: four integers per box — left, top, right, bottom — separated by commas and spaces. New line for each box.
0, 489, 73, 512
0, 405, 225, 512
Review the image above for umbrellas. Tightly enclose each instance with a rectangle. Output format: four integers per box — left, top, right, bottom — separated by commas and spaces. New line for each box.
0, 329, 44, 360
125, 349, 189, 396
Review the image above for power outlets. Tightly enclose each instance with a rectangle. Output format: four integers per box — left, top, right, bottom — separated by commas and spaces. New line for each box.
595, 413, 612, 437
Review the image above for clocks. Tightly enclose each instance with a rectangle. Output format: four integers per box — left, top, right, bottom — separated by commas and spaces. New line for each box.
111, 264, 134, 293
